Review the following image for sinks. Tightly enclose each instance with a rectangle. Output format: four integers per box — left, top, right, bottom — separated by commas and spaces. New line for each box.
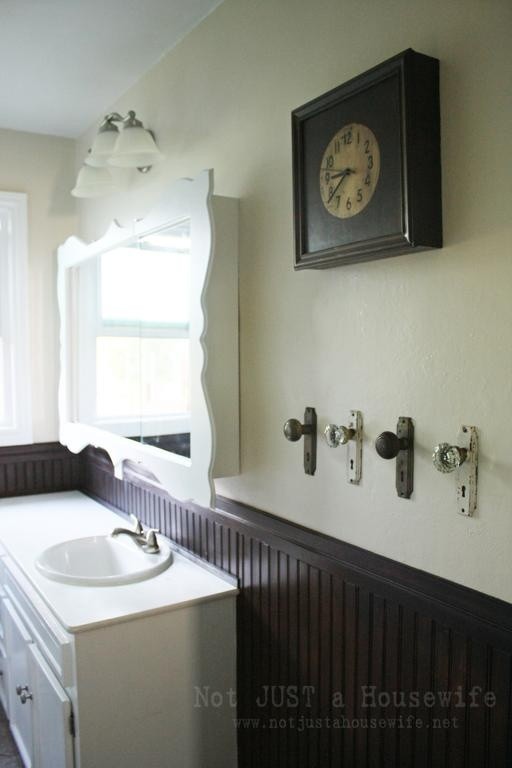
34, 533, 173, 587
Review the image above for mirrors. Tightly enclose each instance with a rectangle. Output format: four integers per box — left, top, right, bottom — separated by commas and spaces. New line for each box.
60, 210, 203, 459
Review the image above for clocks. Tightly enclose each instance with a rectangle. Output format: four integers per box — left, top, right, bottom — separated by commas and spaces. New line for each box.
290, 47, 443, 274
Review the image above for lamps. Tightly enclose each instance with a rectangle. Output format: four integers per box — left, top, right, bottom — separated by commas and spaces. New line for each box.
71, 110, 165, 200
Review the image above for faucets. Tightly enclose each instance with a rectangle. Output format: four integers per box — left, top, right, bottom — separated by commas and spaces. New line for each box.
111, 528, 148, 547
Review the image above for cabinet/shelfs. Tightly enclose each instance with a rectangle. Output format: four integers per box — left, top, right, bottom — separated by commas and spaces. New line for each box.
0, 549, 242, 768
